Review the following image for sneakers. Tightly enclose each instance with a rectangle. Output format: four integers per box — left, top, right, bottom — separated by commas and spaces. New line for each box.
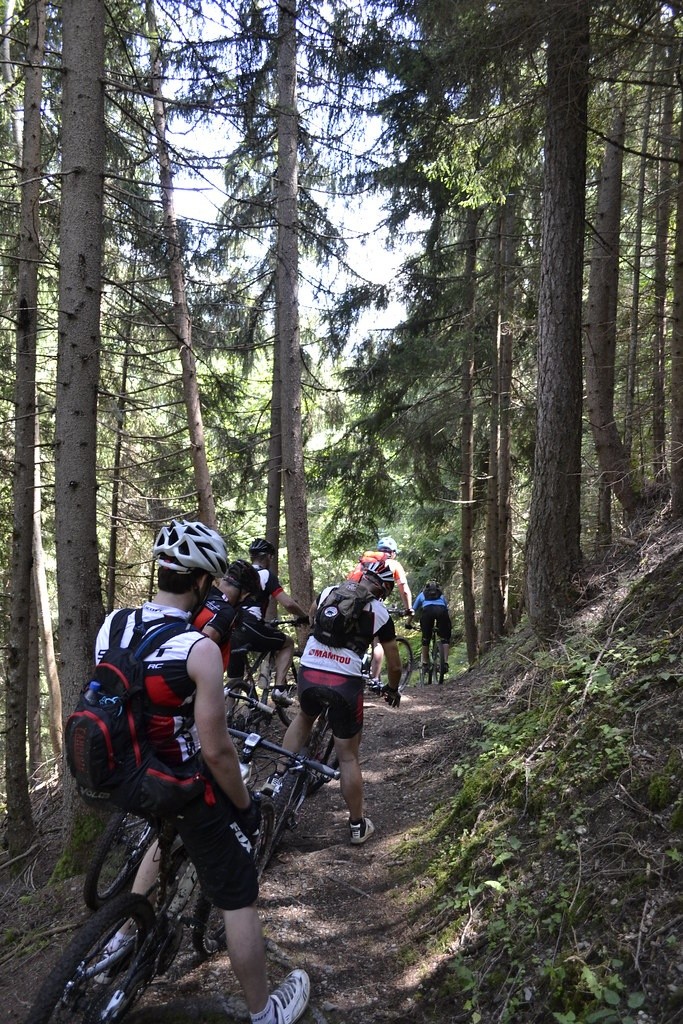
92, 941, 133, 985
348, 817, 375, 844
260, 775, 278, 796
270, 969, 310, 1024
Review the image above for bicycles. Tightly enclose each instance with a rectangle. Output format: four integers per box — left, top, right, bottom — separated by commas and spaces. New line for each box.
410, 625, 446, 685
359, 604, 414, 693
24, 615, 389, 1024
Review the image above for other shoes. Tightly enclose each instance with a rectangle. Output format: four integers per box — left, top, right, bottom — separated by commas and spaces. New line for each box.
368, 679, 385, 696
273, 689, 294, 705
418, 662, 430, 669
443, 663, 449, 673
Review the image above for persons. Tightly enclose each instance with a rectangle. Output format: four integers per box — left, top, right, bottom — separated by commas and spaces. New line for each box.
193, 559, 260, 673
94, 519, 311, 1024
352, 537, 413, 684
226, 538, 308, 720
405, 579, 452, 666
260, 561, 402, 846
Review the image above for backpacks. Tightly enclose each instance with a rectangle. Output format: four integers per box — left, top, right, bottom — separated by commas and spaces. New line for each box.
422, 585, 443, 602
313, 580, 374, 659
361, 548, 392, 570
65, 612, 207, 812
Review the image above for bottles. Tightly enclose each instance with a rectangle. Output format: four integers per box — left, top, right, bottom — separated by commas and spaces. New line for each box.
258, 660, 269, 689
83, 681, 101, 706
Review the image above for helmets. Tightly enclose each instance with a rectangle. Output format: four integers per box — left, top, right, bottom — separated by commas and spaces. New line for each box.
359, 562, 394, 598
224, 559, 263, 596
377, 536, 399, 555
152, 519, 227, 579
247, 539, 277, 559
427, 578, 439, 587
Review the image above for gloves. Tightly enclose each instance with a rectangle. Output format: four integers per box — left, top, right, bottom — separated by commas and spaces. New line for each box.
380, 685, 401, 710
